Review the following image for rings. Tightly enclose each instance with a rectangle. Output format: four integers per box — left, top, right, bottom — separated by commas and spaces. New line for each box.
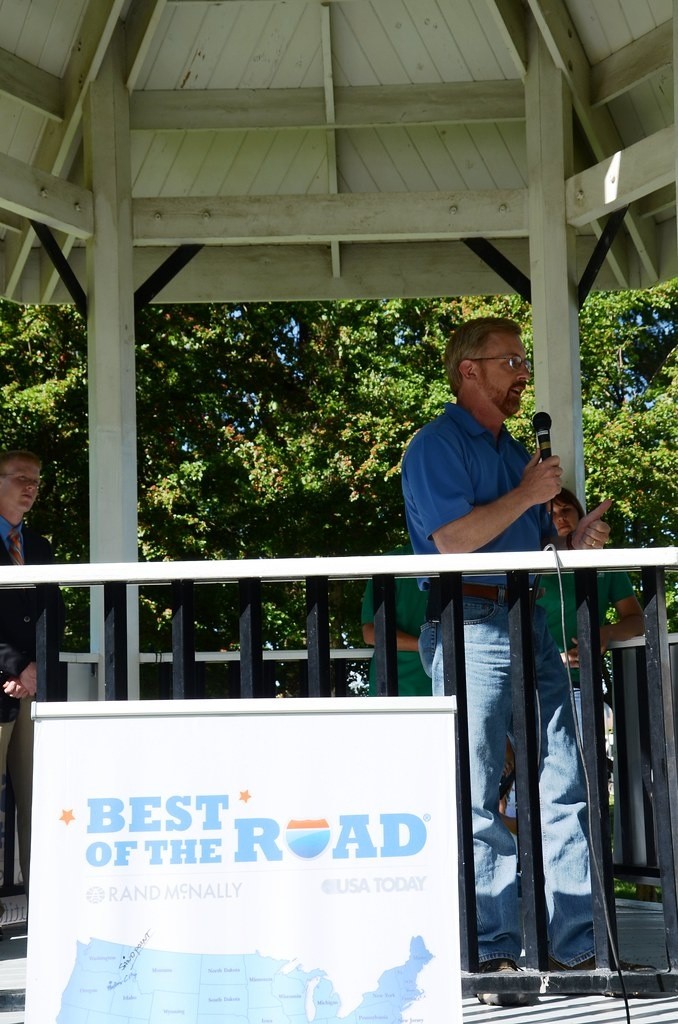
591, 539, 596, 547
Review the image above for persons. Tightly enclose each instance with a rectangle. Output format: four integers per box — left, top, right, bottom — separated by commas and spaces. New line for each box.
402, 318, 666, 1010
362, 547, 432, 697
0, 450, 67, 910
532, 487, 648, 683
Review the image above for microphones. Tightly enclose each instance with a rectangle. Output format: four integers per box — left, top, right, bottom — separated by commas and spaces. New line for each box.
532, 412, 552, 462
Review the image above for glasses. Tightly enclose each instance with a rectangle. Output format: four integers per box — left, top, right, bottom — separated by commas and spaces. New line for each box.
471, 355, 532, 372
0, 472, 41, 487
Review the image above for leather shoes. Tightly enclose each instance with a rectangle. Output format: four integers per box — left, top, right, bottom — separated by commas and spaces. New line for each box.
474, 953, 658, 1009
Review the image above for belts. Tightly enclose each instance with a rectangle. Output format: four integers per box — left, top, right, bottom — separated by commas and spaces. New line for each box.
460, 581, 546, 604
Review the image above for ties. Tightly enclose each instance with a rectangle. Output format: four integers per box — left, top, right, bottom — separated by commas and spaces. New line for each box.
6, 526, 24, 565
